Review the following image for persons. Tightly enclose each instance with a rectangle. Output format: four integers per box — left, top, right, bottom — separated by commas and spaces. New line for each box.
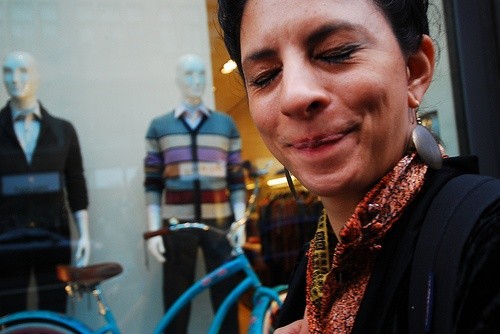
215, 0, 499, 334
0, 50, 91, 317
145, 53, 248, 334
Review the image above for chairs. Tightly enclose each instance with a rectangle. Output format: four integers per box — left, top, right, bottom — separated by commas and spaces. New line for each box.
243, 189, 325, 288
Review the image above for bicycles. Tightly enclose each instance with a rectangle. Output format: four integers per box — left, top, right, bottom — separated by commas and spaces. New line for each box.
0, 159, 289, 334
